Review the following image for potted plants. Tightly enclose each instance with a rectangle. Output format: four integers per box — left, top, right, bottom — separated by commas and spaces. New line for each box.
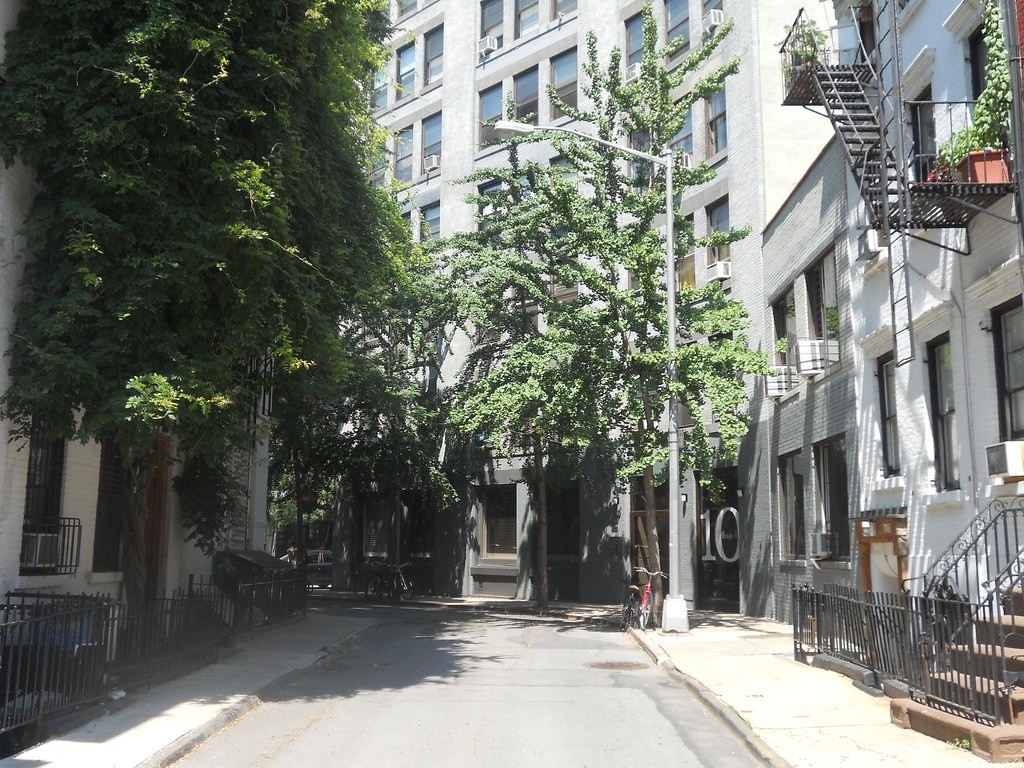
772, 18, 831, 93
935, 1, 1011, 185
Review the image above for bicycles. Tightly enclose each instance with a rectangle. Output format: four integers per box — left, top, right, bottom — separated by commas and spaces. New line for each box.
620, 566, 668, 632
351, 562, 414, 603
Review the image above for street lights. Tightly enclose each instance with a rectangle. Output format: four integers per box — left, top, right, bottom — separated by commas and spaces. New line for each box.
494, 119, 690, 634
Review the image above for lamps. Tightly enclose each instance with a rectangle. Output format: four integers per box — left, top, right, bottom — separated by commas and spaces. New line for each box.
681, 494, 687, 501
735, 489, 744, 498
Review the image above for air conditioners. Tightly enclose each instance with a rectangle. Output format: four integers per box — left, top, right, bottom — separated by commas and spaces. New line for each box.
701, 9, 723, 36
424, 154, 440, 172
764, 366, 799, 399
706, 260, 731, 283
795, 338, 841, 378
477, 36, 498, 57
18, 532, 59, 568
680, 153, 692, 168
625, 62, 639, 86
985, 439, 1024, 480
810, 531, 839, 556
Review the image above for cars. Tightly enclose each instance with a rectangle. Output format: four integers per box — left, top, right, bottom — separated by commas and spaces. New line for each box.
306, 550, 335, 589
